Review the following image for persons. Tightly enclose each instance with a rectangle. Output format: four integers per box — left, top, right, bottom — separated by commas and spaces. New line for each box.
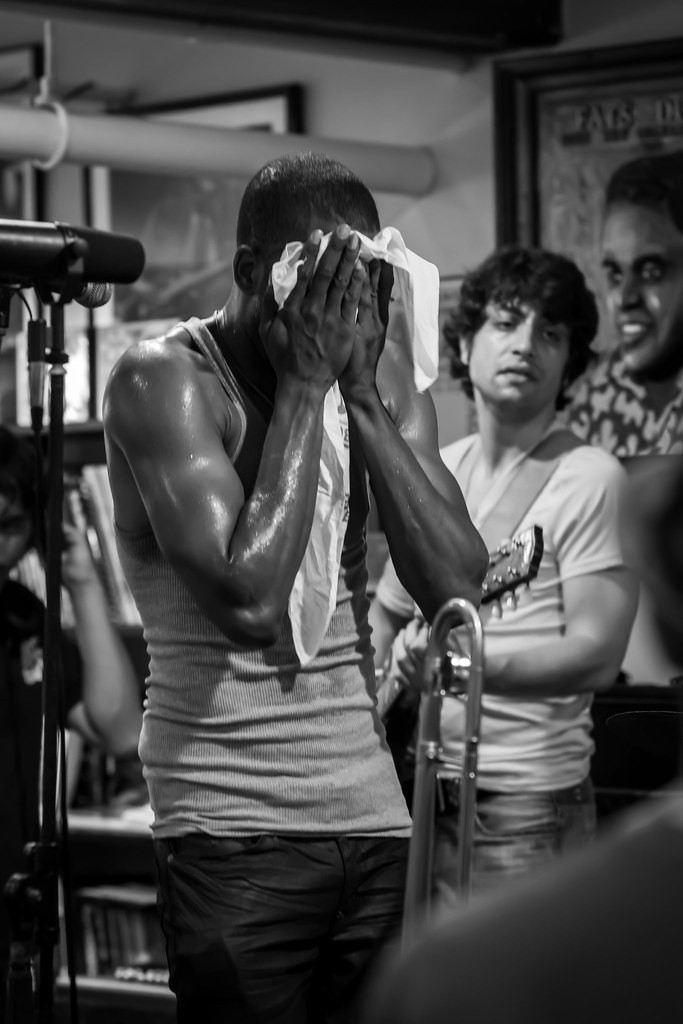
102, 151, 488, 1024
556, 153, 683, 686
367, 245, 641, 919
0, 426, 143, 902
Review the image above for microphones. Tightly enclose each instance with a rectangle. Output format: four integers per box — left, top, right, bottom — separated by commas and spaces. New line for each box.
0, 218, 146, 282
72, 283, 111, 308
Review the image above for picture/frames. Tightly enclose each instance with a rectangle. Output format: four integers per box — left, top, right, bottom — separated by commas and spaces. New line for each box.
79, 84, 307, 417
486, 36, 683, 259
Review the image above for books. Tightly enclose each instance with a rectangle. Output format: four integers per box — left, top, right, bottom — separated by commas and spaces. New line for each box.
33, 704, 152, 821
57, 888, 170, 983
16, 462, 143, 626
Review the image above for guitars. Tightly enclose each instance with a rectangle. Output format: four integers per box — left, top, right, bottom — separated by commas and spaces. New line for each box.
374, 524, 545, 721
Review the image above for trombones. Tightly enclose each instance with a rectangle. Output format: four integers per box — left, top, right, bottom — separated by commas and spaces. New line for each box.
402, 598, 485, 935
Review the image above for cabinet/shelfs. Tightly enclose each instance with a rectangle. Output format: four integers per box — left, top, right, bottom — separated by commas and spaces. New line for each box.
51, 611, 180, 997
